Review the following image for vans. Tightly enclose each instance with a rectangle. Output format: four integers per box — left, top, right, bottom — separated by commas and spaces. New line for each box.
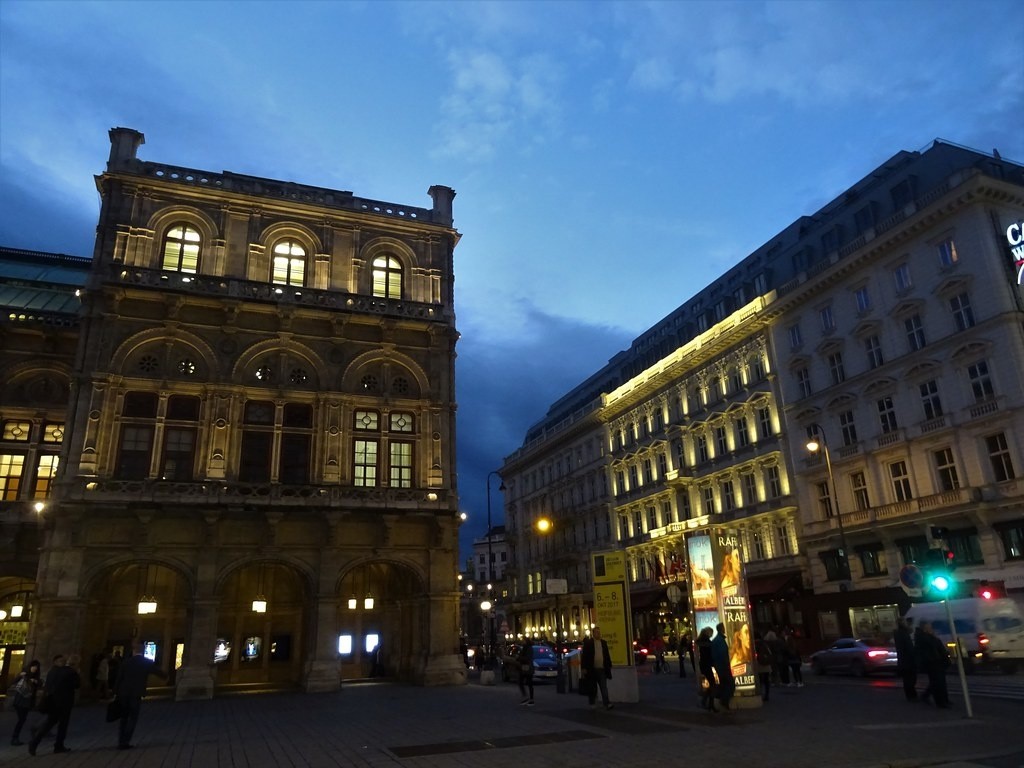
890, 597, 1024, 674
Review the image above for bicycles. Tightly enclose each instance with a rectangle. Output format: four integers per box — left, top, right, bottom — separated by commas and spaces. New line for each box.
651, 652, 671, 674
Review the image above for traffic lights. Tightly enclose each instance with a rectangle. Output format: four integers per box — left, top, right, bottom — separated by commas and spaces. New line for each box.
926, 548, 950, 594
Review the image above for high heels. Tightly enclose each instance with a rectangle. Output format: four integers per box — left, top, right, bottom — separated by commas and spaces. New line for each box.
708, 704, 719, 713
701, 699, 706, 707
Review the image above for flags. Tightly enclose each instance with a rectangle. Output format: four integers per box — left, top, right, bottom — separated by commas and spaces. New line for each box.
647, 549, 685, 584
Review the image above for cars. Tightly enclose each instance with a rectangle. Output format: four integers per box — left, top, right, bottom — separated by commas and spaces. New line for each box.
633, 642, 647, 664
811, 637, 899, 680
501, 645, 559, 685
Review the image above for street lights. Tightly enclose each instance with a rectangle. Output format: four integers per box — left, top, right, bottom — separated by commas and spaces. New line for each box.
806, 423, 851, 580
487, 471, 507, 671
537, 519, 565, 693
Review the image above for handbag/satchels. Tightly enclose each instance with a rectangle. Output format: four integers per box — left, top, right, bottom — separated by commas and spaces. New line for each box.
107, 697, 120, 723
16, 676, 33, 699
37, 691, 52, 715
578, 674, 592, 696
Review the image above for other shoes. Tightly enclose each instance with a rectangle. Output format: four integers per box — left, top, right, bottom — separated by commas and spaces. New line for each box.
796, 682, 804, 688
787, 683, 795, 687
526, 700, 535, 706
588, 705, 596, 710
10, 739, 24, 745
606, 704, 614, 710
54, 747, 71, 753
119, 743, 132, 749
29, 741, 38, 756
779, 682, 785, 686
520, 697, 529, 704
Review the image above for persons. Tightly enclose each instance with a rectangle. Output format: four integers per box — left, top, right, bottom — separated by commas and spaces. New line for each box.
894, 616, 955, 709
720, 543, 755, 684
113, 642, 170, 751
757, 624, 804, 702
12, 647, 125, 756
458, 622, 735, 712
